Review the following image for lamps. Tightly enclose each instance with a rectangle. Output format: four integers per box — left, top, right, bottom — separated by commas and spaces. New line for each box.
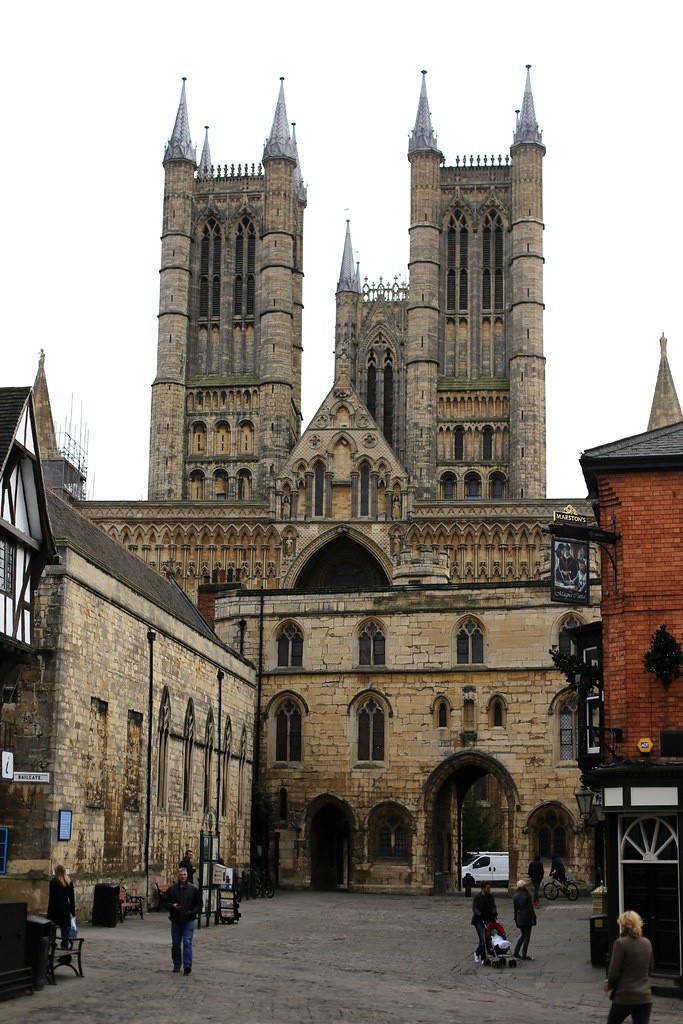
459, 729, 477, 749
573, 781, 606, 835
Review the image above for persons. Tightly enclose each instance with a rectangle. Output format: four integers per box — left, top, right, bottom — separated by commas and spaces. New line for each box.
528, 855, 545, 906
604, 911, 654, 1024
179, 850, 196, 883
513, 880, 537, 960
47, 865, 76, 948
162, 867, 203, 974
472, 881, 511, 965
548, 856, 567, 895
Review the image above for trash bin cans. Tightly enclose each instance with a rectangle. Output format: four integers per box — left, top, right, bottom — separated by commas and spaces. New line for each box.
25, 915, 54, 989
590, 914, 609, 967
93, 884, 122, 928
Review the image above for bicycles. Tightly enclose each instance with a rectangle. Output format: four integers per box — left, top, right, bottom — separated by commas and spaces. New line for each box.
248, 867, 275, 898
543, 872, 579, 902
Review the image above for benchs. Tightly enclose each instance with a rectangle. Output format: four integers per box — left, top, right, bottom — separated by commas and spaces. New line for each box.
154, 882, 171, 911
119, 887, 145, 923
47, 927, 85, 985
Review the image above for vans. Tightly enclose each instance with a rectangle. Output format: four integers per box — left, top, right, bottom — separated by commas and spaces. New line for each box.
461, 850, 509, 888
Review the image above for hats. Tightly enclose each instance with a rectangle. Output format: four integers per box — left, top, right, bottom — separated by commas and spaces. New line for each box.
517, 880, 526, 888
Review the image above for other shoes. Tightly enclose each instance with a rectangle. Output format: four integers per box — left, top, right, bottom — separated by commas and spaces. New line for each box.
482, 960, 484, 964
514, 954, 522, 958
473, 952, 480, 962
184, 967, 192, 975
173, 968, 180, 973
534, 903, 540, 908
523, 956, 535, 961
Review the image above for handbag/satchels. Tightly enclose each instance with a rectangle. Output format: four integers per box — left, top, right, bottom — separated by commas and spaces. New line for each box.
68, 917, 78, 944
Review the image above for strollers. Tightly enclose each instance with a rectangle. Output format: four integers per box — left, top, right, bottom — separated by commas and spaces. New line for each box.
482, 918, 517, 968
